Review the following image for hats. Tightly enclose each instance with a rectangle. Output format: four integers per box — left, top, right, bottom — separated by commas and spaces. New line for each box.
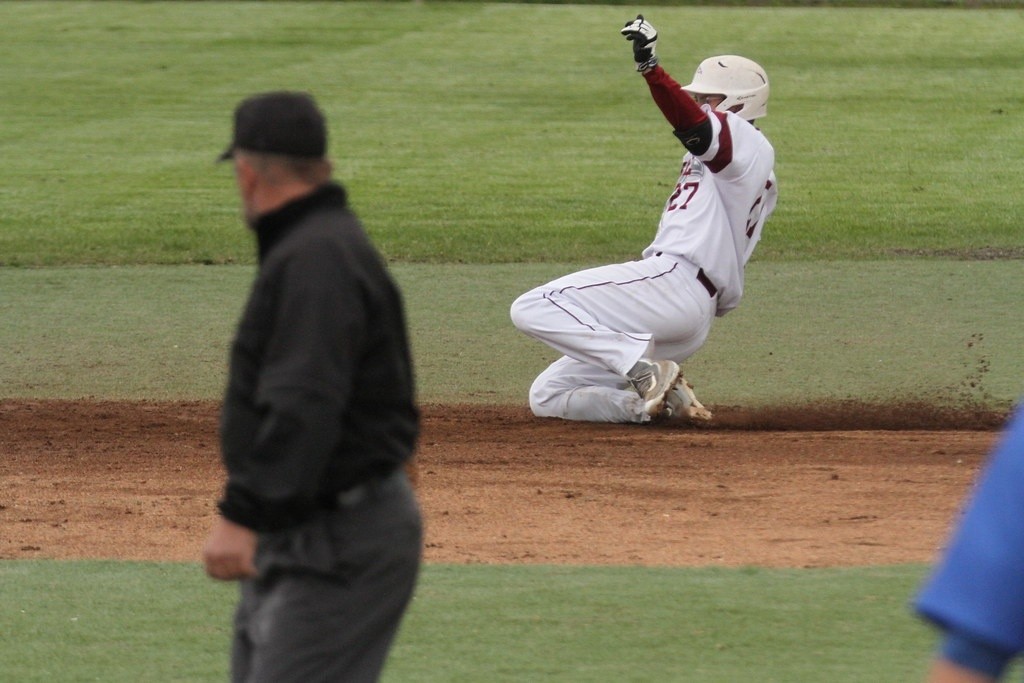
219, 92, 327, 159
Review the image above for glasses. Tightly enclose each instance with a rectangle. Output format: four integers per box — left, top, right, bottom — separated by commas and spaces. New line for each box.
691, 96, 726, 103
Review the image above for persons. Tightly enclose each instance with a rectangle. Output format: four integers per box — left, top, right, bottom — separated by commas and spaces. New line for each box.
910, 400, 1024, 683
510, 14, 778, 427
203, 90, 423, 683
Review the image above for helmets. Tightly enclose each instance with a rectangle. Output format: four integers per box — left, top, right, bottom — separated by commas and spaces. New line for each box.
680, 54, 770, 121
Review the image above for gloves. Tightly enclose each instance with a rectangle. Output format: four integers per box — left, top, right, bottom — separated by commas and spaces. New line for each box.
620, 15, 658, 71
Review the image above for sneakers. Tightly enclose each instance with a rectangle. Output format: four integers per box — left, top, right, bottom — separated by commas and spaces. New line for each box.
660, 375, 715, 426
624, 360, 680, 416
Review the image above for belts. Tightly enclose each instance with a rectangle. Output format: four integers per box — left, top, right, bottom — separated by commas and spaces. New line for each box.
656, 251, 718, 298
343, 477, 375, 506
